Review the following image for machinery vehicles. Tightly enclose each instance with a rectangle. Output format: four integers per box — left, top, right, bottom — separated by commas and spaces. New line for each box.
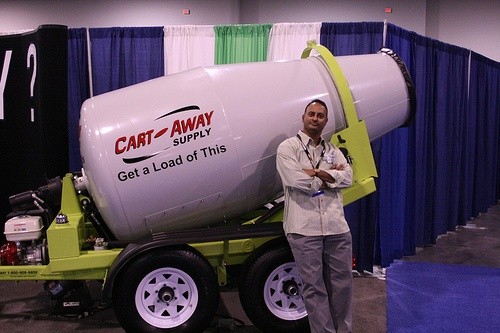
0, 41, 418, 333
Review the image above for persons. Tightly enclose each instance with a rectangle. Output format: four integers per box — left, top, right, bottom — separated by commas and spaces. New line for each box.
274, 98, 354, 333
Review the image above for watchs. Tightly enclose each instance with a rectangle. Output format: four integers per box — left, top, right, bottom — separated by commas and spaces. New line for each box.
315, 168, 320, 177
